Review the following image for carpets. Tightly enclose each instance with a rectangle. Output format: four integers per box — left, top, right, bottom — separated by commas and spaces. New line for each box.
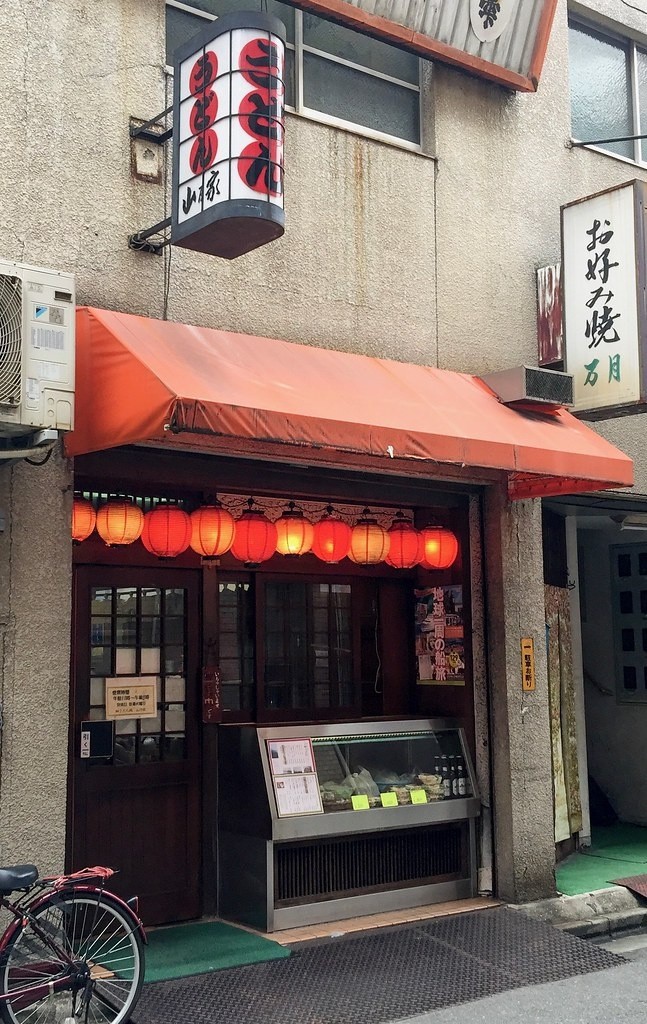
555, 821, 647, 895
63, 921, 291, 985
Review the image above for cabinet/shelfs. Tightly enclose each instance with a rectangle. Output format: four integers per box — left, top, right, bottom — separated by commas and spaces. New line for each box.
218, 715, 481, 842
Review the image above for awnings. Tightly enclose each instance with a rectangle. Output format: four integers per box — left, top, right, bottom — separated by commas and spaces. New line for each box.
58, 304, 635, 505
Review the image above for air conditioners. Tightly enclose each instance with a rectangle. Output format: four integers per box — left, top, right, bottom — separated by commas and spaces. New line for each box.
0, 260, 76, 438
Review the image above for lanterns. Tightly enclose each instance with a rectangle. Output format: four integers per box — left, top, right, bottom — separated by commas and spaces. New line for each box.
229, 496, 279, 569
384, 509, 425, 570
346, 504, 391, 567
272, 500, 315, 559
139, 496, 193, 562
95, 491, 145, 550
188, 492, 237, 570
311, 503, 352, 567
417, 516, 459, 571
71, 490, 97, 546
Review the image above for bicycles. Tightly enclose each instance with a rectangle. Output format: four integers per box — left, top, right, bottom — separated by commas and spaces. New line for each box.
0, 861, 150, 1024
